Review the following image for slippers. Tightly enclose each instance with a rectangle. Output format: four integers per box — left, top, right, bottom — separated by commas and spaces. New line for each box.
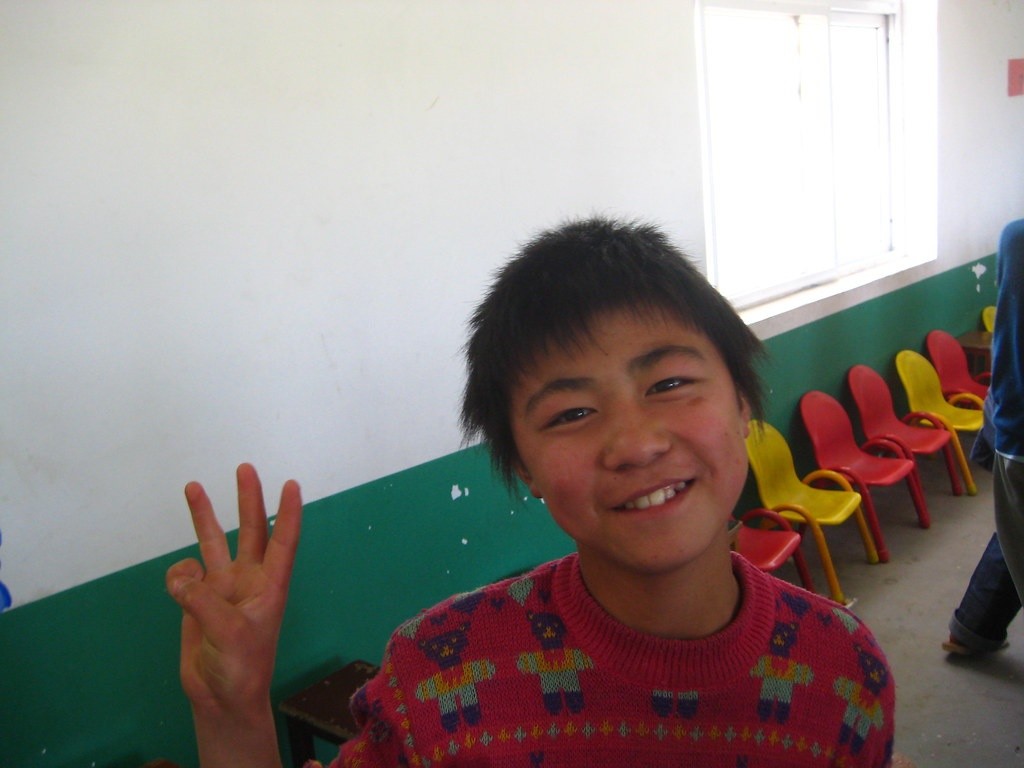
942, 641, 1010, 654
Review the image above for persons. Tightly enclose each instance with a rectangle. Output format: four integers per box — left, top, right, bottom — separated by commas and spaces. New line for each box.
933, 217, 1024, 665
166, 217, 898, 768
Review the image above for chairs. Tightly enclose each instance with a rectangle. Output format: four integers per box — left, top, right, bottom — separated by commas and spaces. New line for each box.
926, 330, 991, 410
744, 419, 879, 606
728, 508, 815, 594
895, 350, 985, 496
801, 390, 930, 562
982, 305, 997, 333
848, 365, 962, 511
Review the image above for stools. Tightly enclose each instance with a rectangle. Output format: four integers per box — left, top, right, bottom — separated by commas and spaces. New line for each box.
281, 660, 382, 768
954, 332, 993, 373
